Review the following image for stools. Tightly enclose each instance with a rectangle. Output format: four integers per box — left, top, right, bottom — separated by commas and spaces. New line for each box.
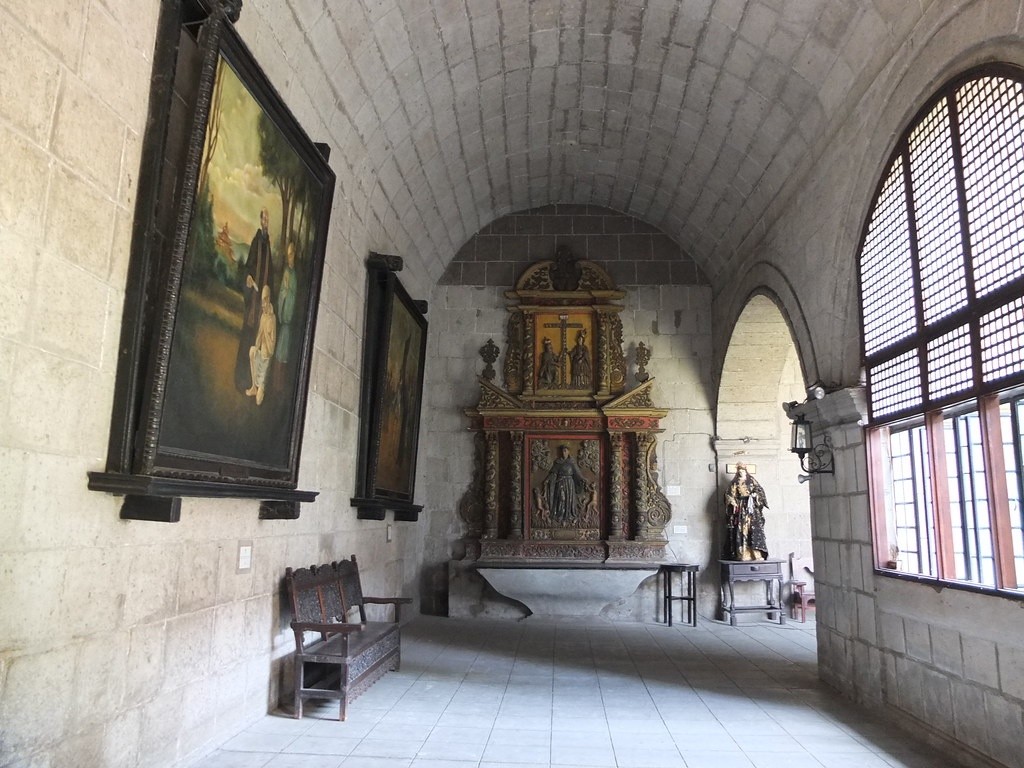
661, 563, 700, 627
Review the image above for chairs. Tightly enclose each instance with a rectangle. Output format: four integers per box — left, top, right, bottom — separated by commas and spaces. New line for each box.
788, 552, 815, 623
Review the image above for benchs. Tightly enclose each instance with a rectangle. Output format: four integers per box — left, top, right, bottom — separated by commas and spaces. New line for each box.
285, 554, 414, 722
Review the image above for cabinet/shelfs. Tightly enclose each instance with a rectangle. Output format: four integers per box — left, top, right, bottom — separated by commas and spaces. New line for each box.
718, 557, 787, 626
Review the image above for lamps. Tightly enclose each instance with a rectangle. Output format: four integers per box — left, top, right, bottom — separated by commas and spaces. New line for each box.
792, 412, 836, 484
803, 385, 825, 405
781, 400, 799, 412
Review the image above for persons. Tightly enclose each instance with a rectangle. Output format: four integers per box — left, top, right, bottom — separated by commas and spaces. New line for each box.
566, 330, 591, 387
535, 445, 599, 522
724, 462, 769, 560
540, 340, 563, 387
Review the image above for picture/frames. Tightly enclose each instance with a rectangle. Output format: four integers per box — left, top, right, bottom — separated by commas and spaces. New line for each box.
89, 0, 336, 523
350, 251, 429, 522
